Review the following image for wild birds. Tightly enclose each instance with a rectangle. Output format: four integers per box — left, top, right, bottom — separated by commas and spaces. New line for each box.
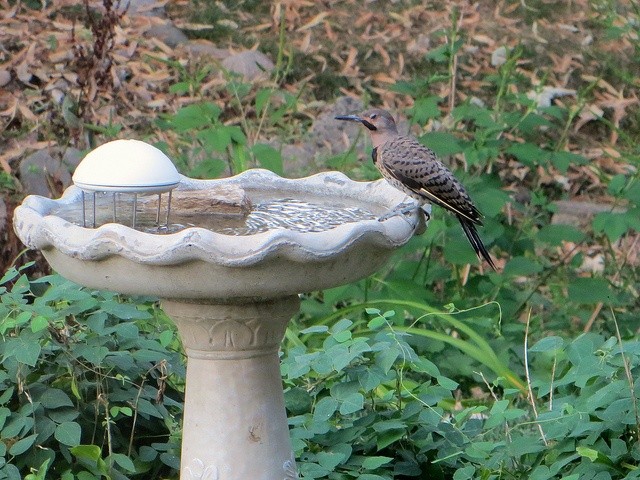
334, 109, 499, 275
